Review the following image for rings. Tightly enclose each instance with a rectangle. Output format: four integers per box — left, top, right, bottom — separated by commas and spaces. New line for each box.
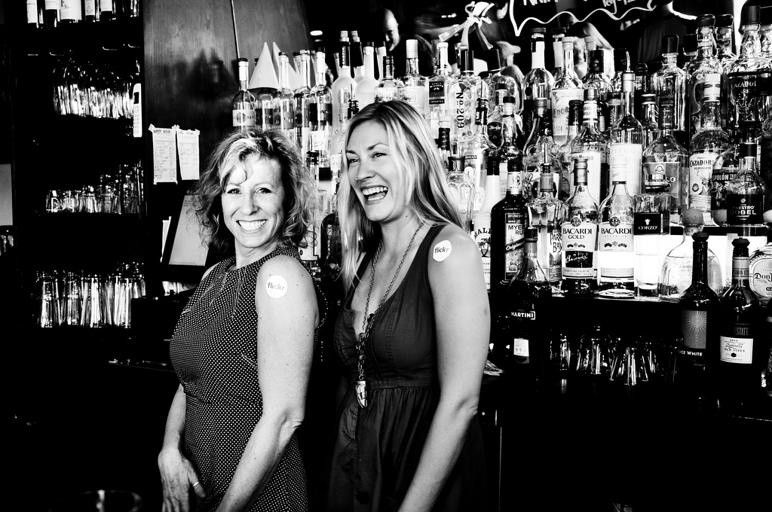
193, 482, 199, 487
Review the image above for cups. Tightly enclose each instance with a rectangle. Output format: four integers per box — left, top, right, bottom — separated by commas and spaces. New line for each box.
544, 325, 659, 388
40, 261, 147, 333
43, 159, 148, 212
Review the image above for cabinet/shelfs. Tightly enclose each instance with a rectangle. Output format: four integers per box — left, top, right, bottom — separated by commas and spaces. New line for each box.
26, 39, 152, 341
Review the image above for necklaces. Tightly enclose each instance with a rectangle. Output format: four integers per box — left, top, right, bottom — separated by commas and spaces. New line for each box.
354, 219, 425, 409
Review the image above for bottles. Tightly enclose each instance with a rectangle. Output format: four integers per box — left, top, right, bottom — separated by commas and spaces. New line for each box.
298, 158, 341, 264
721, 238, 761, 371
504, 227, 545, 384
225, 18, 427, 160
427, 18, 772, 302
673, 232, 722, 375
22, 0, 143, 142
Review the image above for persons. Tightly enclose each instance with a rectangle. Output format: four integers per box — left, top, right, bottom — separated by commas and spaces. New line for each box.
325, 100, 492, 512
157, 129, 331, 512
559, 7, 614, 80
367, 8, 434, 79
